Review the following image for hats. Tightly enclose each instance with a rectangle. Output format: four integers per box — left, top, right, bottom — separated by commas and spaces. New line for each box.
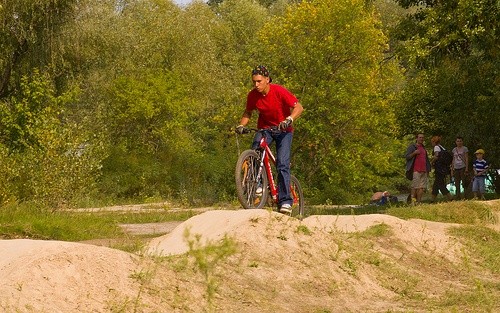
252, 65, 272, 83
474, 148, 485, 154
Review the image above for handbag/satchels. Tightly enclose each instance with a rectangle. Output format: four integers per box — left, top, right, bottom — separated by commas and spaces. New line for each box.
433, 146, 453, 166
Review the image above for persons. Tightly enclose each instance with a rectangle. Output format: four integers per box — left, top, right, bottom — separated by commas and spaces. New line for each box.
472, 149, 489, 201
235, 66, 304, 213
404, 132, 431, 207
429, 136, 454, 203
450, 136, 469, 201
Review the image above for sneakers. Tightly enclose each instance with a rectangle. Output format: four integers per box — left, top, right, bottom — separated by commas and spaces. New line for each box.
280, 203, 292, 213
255, 185, 270, 196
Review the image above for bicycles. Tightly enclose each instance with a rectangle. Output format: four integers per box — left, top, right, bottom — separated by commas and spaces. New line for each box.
235, 124, 304, 221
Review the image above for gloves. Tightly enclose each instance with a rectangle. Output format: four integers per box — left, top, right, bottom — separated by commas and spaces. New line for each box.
278, 116, 292, 131
235, 126, 249, 135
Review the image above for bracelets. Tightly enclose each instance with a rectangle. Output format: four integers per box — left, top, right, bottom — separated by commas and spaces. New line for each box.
288, 116, 294, 123
